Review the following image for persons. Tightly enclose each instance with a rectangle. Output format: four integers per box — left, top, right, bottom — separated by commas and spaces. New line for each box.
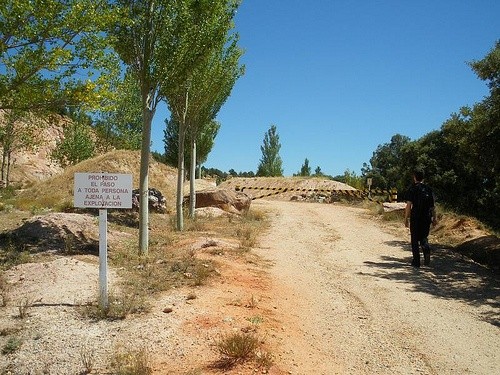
404, 167, 437, 268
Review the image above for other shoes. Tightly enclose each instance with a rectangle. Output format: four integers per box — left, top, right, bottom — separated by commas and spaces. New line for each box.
424, 259, 430, 264
412, 260, 420, 266
